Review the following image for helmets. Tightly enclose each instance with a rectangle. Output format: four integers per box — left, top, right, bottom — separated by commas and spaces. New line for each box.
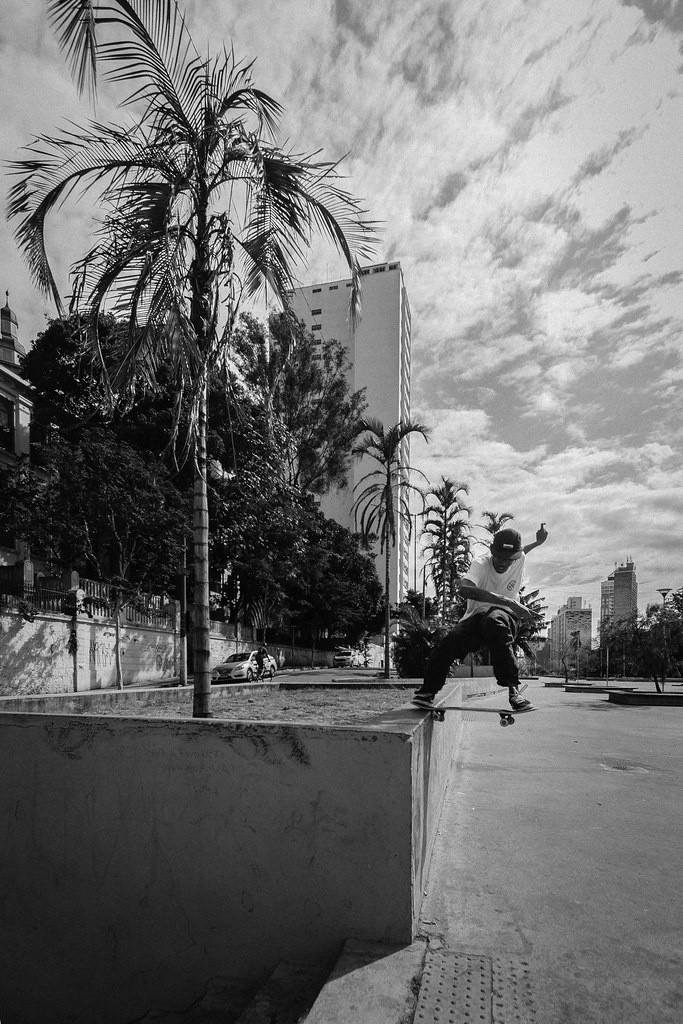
261, 643, 267, 647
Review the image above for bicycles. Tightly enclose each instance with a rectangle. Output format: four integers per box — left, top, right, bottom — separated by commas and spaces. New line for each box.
249, 664, 273, 684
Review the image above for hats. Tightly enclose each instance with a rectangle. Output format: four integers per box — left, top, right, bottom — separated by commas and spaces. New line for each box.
491, 528, 522, 559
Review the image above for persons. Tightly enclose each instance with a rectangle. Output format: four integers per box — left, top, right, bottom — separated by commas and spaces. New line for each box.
255, 643, 271, 680
412, 522, 549, 712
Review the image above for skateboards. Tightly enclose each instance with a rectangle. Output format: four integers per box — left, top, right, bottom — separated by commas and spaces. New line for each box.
410, 701, 538, 727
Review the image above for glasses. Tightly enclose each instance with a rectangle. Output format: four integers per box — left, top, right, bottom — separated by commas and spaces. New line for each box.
491, 556, 518, 564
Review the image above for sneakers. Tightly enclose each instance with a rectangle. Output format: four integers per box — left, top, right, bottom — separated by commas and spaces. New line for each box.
410, 689, 435, 708
509, 683, 534, 711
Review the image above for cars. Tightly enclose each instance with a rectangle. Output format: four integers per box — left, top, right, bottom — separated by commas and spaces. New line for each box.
212, 650, 277, 683
332, 650, 360, 669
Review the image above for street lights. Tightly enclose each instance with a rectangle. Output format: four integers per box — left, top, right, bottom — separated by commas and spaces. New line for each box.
603, 614, 614, 686
656, 588, 672, 693
